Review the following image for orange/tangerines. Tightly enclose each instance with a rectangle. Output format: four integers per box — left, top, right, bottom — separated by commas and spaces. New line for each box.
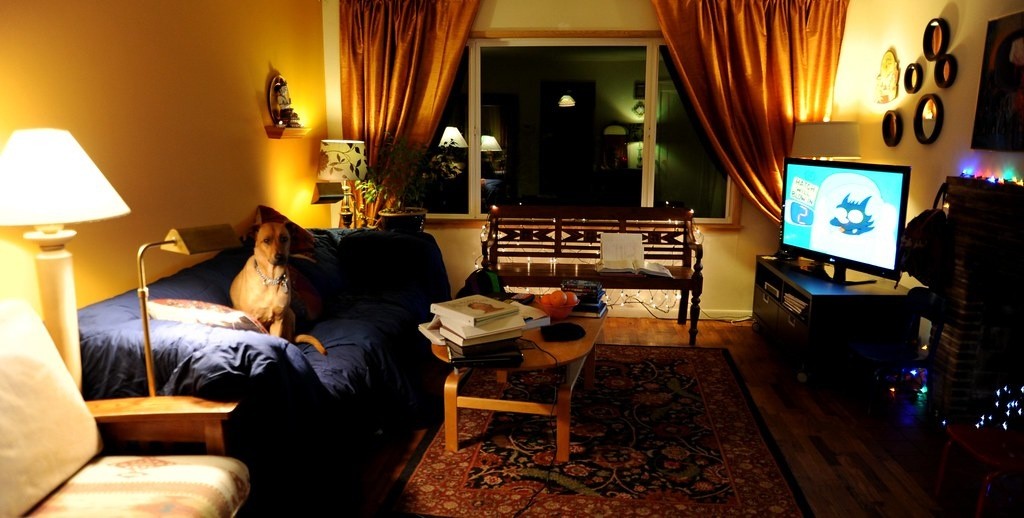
541, 290, 578, 318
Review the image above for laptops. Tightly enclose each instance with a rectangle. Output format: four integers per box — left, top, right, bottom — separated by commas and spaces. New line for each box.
446, 338, 524, 367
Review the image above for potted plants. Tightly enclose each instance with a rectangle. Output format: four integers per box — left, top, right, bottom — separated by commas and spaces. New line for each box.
366, 135, 461, 234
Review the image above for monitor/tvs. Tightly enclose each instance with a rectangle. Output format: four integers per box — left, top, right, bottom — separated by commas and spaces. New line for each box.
779, 156, 912, 285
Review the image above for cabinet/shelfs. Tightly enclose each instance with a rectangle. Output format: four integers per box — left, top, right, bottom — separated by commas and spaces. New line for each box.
751, 256, 920, 390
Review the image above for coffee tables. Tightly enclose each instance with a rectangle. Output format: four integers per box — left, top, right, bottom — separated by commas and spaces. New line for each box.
443, 305, 607, 464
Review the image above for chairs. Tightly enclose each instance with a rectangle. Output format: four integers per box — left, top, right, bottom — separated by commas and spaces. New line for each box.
0, 296, 247, 518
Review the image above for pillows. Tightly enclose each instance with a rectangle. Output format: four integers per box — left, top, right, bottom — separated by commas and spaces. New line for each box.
252, 206, 314, 254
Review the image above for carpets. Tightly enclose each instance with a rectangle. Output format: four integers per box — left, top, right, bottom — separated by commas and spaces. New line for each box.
371, 348, 815, 518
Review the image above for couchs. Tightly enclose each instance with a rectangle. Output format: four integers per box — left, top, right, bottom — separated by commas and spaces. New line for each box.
76, 225, 452, 518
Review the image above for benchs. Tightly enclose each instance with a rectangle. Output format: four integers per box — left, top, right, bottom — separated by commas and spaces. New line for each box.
480, 205, 703, 345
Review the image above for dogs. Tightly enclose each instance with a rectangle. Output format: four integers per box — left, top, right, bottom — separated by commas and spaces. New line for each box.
230, 219, 328, 356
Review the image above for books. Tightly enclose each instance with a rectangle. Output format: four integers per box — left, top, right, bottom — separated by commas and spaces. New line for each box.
562, 279, 607, 317
418, 294, 550, 368
596, 234, 674, 278
764, 281, 808, 314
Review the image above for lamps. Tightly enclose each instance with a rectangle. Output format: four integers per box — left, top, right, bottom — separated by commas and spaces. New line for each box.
438, 127, 502, 152
136, 222, 246, 395
558, 95, 576, 107
318, 139, 366, 229
0, 129, 129, 400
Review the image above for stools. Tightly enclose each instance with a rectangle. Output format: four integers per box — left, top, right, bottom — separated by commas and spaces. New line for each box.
934, 426, 1024, 518
